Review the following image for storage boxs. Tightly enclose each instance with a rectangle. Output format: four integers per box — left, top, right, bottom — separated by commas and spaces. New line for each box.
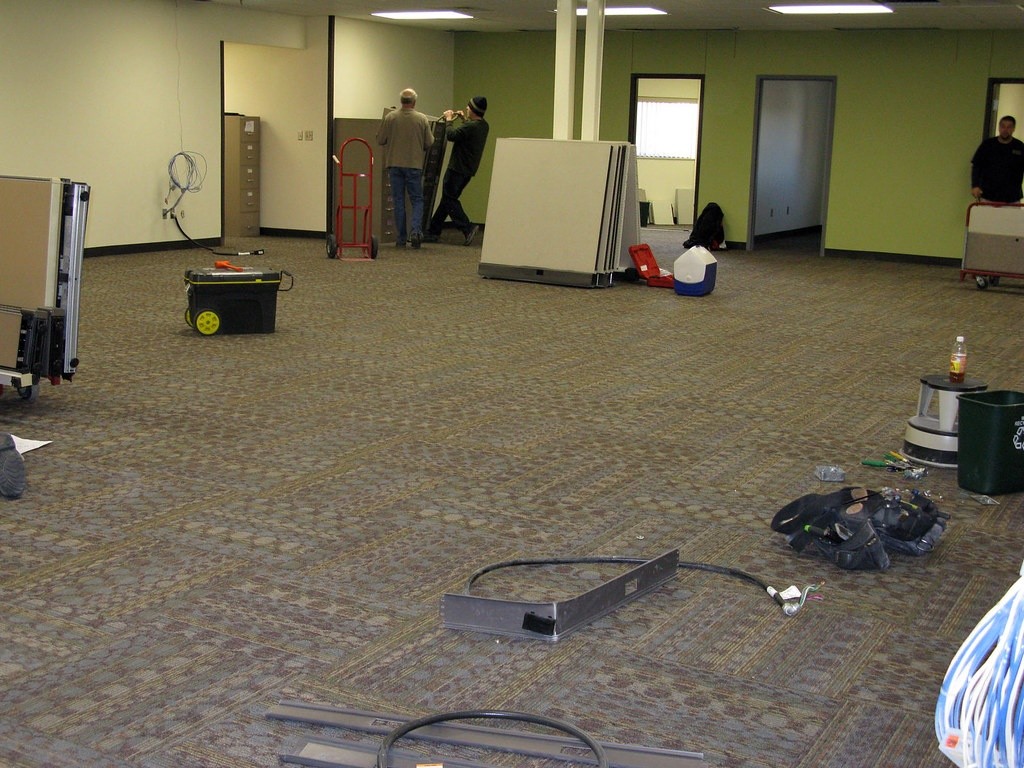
184, 268, 280, 334
383, 108, 448, 241
335, 119, 412, 243
672, 244, 717, 297
627, 244, 674, 288
224, 116, 261, 237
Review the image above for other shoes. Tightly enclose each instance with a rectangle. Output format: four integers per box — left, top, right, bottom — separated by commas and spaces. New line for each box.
411, 232, 421, 250
396, 242, 406, 249
463, 222, 479, 246
421, 231, 440, 241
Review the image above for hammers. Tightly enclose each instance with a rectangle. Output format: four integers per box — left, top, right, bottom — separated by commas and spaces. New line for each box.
214, 259, 242, 271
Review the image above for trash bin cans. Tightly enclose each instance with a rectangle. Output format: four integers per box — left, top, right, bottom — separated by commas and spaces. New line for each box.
954, 388, 1024, 496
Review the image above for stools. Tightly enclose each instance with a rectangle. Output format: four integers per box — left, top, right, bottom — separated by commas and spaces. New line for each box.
899, 374, 988, 471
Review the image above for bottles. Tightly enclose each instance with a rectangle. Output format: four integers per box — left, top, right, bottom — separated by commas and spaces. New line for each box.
949, 336, 967, 383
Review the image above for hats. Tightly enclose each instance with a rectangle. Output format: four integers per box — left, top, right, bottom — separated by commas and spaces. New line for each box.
468, 96, 487, 117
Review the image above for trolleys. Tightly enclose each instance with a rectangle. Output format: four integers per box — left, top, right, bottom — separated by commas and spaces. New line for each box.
0, 369, 38, 403
326, 138, 379, 261
422, 112, 459, 243
969, 193, 1024, 289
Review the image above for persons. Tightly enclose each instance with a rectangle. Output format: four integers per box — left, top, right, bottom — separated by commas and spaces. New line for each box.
971, 116, 1024, 287
423, 96, 490, 246
376, 88, 434, 249
